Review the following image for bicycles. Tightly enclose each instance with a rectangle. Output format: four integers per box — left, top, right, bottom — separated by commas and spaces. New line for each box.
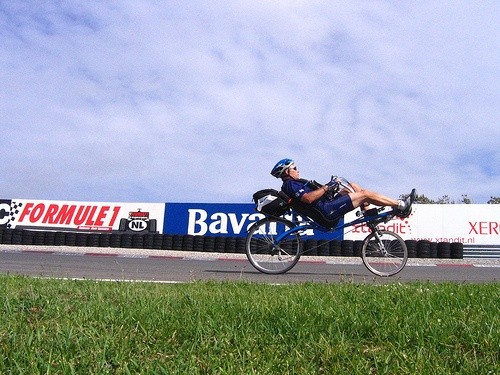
244, 175, 412, 277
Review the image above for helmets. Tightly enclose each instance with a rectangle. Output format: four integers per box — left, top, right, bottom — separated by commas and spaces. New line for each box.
271, 159, 295, 178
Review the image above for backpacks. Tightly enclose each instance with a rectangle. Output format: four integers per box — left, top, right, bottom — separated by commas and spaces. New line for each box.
253, 189, 289, 217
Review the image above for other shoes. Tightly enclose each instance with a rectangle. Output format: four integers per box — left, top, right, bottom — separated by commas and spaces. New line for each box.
395, 188, 416, 218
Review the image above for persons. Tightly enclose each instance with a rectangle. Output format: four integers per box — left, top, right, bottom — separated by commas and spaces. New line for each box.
271, 158, 416, 220
